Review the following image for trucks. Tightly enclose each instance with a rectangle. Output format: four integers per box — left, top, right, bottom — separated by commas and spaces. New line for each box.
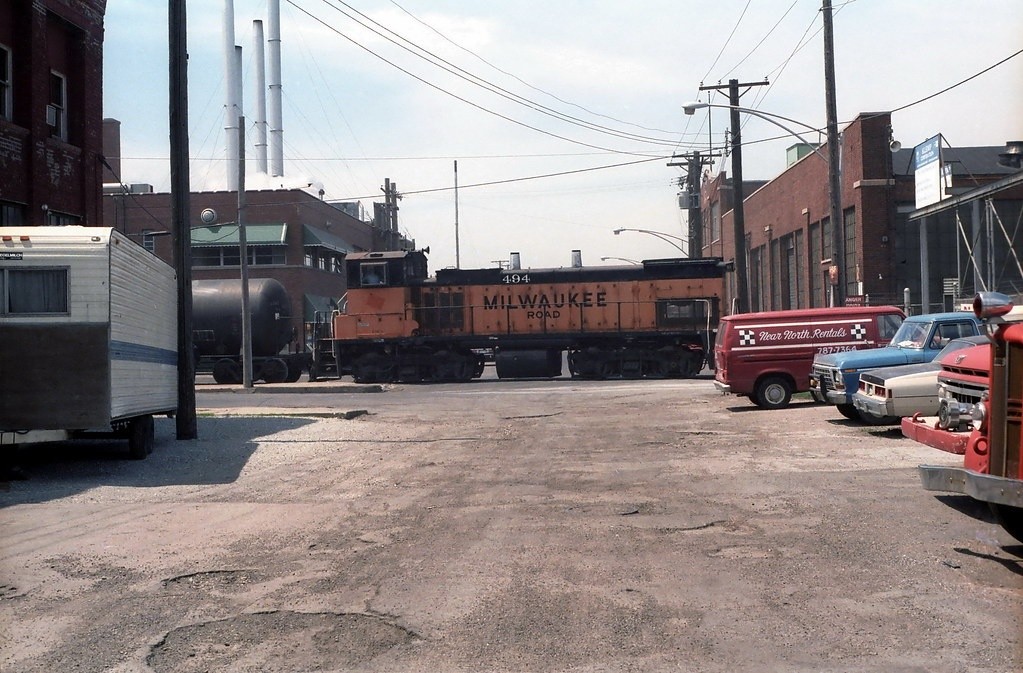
901, 291, 1023, 543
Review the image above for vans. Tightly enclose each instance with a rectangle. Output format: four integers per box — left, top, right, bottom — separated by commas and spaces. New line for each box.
713, 304, 943, 410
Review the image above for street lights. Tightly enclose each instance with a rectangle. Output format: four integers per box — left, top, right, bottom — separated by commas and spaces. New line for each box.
681, 102, 847, 309
613, 227, 694, 260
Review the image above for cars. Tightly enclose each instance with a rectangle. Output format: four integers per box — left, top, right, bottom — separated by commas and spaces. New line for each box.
808, 313, 987, 426
852, 333, 990, 419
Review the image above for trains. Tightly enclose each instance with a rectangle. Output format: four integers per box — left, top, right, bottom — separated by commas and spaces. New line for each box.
191, 245, 735, 386
